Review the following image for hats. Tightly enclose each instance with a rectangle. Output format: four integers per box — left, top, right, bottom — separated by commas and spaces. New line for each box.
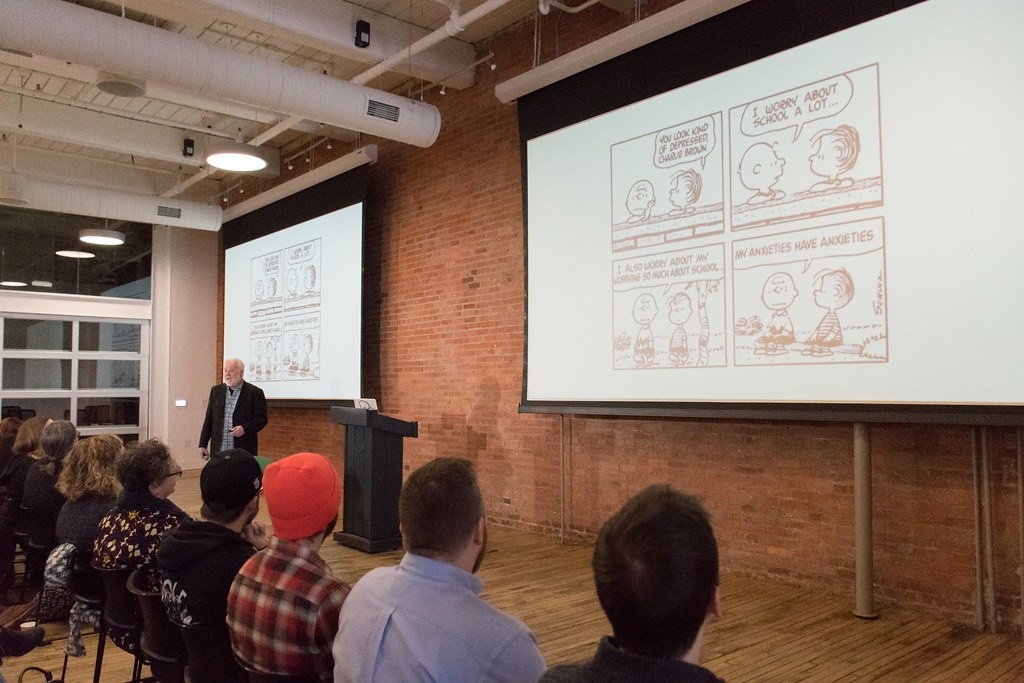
200, 447, 263, 512
262, 450, 342, 542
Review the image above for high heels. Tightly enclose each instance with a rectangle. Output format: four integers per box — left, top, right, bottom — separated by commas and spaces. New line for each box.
0, 624, 46, 665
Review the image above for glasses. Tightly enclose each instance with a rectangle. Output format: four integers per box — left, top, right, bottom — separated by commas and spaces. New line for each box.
158, 465, 183, 481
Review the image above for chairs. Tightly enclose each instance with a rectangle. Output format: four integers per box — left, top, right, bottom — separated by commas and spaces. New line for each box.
1, 483, 322, 683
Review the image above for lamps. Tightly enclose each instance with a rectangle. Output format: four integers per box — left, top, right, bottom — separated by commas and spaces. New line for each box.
0, 250, 96, 288
79, 227, 127, 246
206, 141, 270, 174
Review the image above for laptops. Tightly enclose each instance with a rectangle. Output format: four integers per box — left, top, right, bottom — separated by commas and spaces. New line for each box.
354, 398, 378, 410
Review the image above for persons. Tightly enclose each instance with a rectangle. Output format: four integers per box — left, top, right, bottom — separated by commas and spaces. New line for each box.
198, 358, 268, 460
254, 265, 316, 372
0, 416, 727, 683
627, 126, 860, 365
